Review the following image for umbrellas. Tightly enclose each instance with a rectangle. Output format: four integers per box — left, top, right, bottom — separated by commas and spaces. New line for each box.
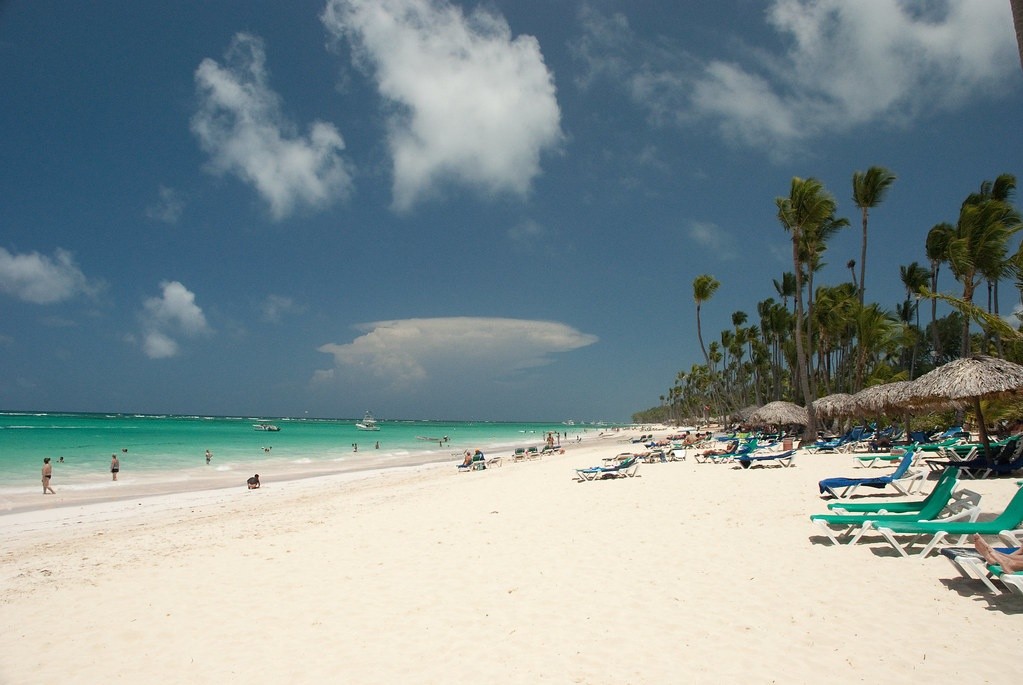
751, 400, 809, 440
804, 393, 855, 435
845, 384, 891, 441
729, 405, 763, 430
897, 354, 1023, 453
859, 380, 972, 445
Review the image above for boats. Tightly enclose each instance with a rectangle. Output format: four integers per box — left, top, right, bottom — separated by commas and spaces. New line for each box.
355, 411, 381, 432
252, 424, 280, 432
417, 434, 447, 442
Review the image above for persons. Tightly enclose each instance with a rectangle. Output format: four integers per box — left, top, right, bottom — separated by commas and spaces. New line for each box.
838, 434, 889, 452
974, 532, 1023, 574
547, 433, 560, 448
653, 440, 669, 446
564, 431, 567, 438
576, 435, 581, 442
462, 451, 472, 468
542, 430, 546, 441
701, 442, 735, 457
728, 425, 738, 438
682, 430, 711, 448
638, 434, 652, 439
474, 449, 486, 469
56, 433, 452, 489
928, 431, 944, 440
615, 452, 649, 459
41, 457, 55, 493
667, 433, 686, 439
524, 426, 667, 432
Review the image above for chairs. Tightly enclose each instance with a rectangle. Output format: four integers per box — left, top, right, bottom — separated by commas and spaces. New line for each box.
483, 456, 504, 468
552, 445, 563, 453
525, 446, 543, 461
458, 455, 485, 473
573, 422, 1023, 596
540, 445, 555, 457
512, 447, 526, 462
